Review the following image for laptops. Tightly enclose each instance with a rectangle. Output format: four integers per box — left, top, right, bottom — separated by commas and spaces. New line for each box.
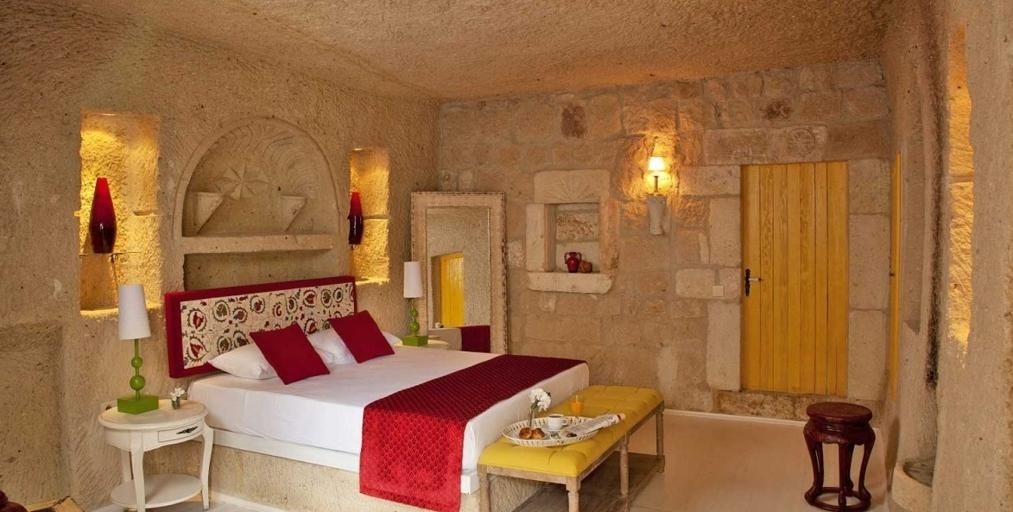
531, 428, 544, 439
519, 427, 532, 439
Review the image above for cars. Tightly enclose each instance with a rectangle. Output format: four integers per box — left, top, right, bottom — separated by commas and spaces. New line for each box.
170, 397, 181, 409
546, 413, 568, 429
569, 394, 585, 416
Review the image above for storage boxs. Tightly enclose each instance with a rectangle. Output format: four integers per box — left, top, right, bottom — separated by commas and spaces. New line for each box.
479, 384, 665, 512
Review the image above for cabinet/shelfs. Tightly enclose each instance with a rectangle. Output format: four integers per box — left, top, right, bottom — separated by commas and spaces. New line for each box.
563, 251, 582, 273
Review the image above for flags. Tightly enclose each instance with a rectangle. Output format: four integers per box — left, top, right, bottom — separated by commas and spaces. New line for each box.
562, 250, 583, 273
172, 398, 180, 410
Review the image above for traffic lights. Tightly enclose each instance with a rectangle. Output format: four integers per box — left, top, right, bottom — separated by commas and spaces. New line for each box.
330, 310, 395, 364
207, 341, 336, 379
250, 320, 330, 385
309, 327, 400, 367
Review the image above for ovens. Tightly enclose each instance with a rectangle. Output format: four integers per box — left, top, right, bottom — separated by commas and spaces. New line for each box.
163, 275, 591, 511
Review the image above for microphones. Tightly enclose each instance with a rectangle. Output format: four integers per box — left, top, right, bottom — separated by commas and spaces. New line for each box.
803, 402, 875, 512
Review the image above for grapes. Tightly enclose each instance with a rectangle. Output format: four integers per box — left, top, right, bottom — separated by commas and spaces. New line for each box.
397, 338, 452, 349
98, 399, 214, 512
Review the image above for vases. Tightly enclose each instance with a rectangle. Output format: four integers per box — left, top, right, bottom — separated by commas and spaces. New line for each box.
169, 387, 185, 402
529, 388, 552, 427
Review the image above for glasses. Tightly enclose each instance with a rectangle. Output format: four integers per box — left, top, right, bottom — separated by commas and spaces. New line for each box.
648, 156, 664, 196
117, 283, 158, 413
350, 192, 364, 244
402, 261, 428, 346
89, 176, 116, 253
